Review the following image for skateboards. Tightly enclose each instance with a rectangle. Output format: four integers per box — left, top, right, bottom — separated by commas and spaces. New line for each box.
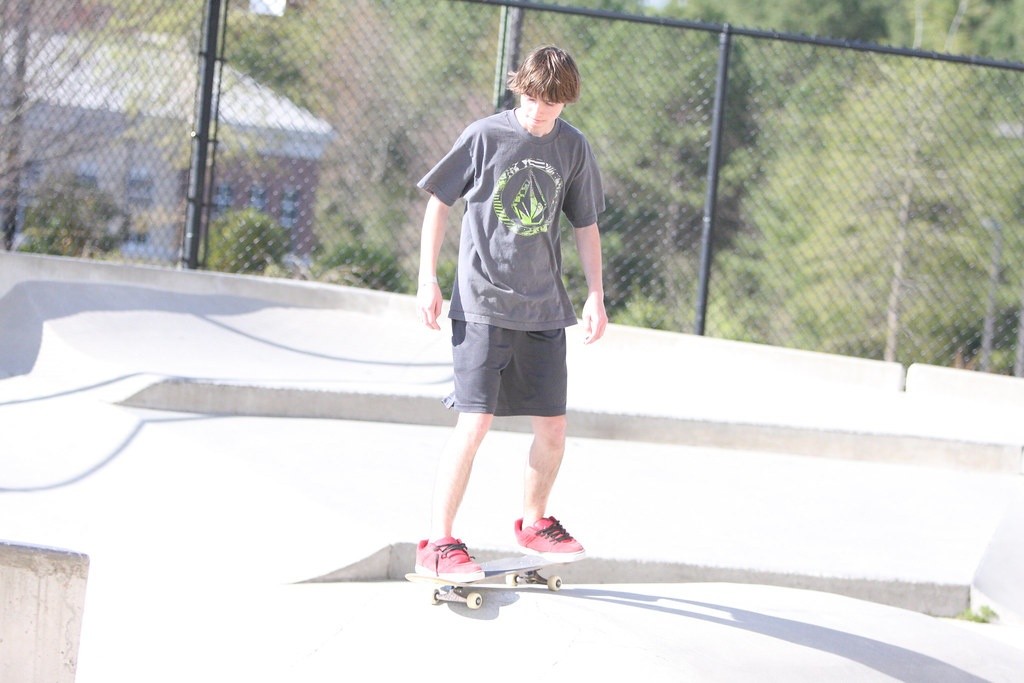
403, 550, 590, 612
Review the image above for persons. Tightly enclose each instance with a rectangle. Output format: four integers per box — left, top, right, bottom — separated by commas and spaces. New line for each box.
415, 46, 609, 584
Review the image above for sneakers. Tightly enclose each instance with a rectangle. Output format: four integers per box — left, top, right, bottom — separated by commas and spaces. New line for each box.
415, 537, 485, 583
516, 516, 584, 557
